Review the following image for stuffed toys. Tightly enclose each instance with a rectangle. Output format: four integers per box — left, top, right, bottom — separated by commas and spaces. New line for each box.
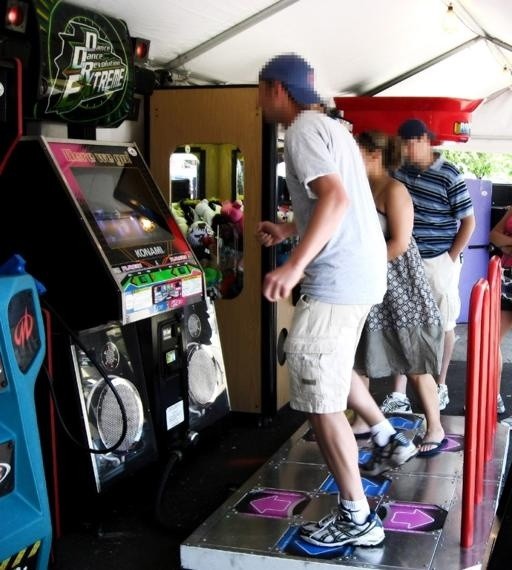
172, 196, 297, 296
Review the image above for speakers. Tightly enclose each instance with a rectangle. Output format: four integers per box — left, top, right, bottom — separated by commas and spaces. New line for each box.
69, 319, 160, 493
181, 297, 233, 433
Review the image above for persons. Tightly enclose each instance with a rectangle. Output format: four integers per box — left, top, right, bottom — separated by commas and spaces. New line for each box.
382, 117, 477, 416
490, 206, 512, 416
245, 51, 416, 547
354, 128, 447, 455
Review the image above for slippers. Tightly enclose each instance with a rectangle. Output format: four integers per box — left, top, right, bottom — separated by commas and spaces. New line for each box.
417, 439, 448, 456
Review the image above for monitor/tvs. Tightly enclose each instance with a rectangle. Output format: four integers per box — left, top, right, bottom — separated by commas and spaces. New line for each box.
47, 141, 194, 269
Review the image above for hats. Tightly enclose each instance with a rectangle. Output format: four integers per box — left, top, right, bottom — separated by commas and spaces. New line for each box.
261, 54, 319, 104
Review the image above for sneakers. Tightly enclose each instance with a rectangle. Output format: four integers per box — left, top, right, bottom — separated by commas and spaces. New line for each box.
359, 432, 419, 477
436, 383, 449, 411
379, 394, 412, 414
299, 504, 385, 547
497, 394, 505, 413
